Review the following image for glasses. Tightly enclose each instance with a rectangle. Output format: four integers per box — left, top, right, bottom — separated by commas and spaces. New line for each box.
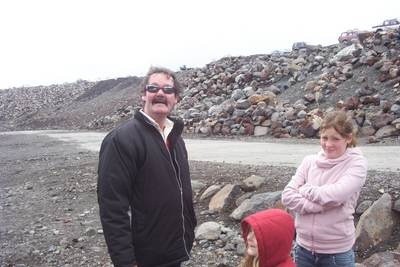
145, 85, 177, 94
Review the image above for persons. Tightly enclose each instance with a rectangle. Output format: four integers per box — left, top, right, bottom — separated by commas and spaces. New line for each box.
97, 64, 198, 267
238, 209, 296, 267
281, 109, 367, 267
